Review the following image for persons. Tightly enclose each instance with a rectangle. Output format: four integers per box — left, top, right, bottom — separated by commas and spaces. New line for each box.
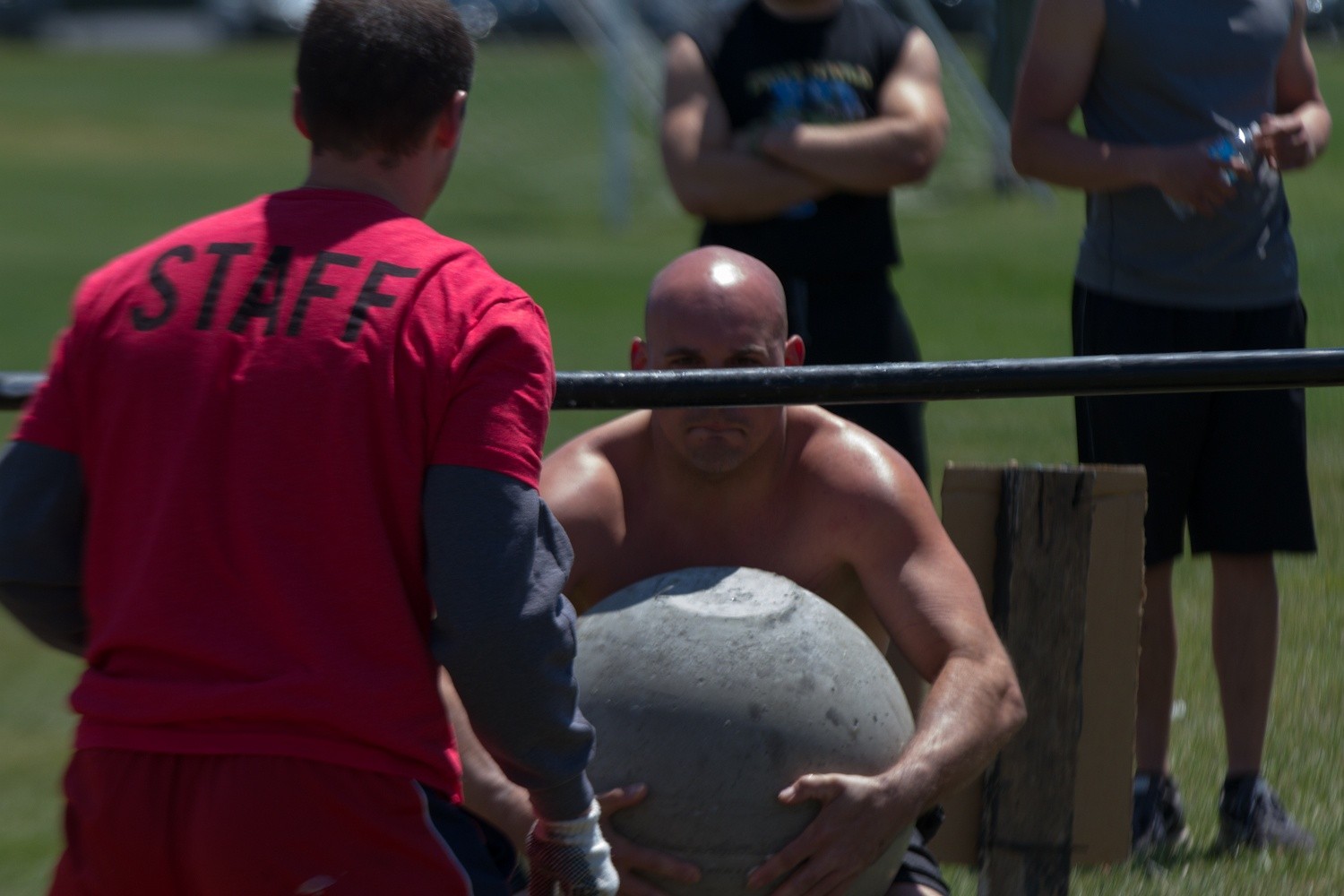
652, 2, 951, 715
0, 1, 620, 896
1010, 0, 1332, 861
437, 243, 1029, 896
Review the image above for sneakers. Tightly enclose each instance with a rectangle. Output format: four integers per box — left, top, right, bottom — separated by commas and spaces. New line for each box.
1132, 773, 1195, 861
1215, 778, 1317, 851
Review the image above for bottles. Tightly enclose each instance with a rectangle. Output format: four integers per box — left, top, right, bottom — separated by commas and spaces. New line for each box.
1163, 121, 1262, 224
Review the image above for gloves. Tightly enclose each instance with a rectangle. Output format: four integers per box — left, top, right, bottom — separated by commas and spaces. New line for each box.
526, 798, 620, 896
